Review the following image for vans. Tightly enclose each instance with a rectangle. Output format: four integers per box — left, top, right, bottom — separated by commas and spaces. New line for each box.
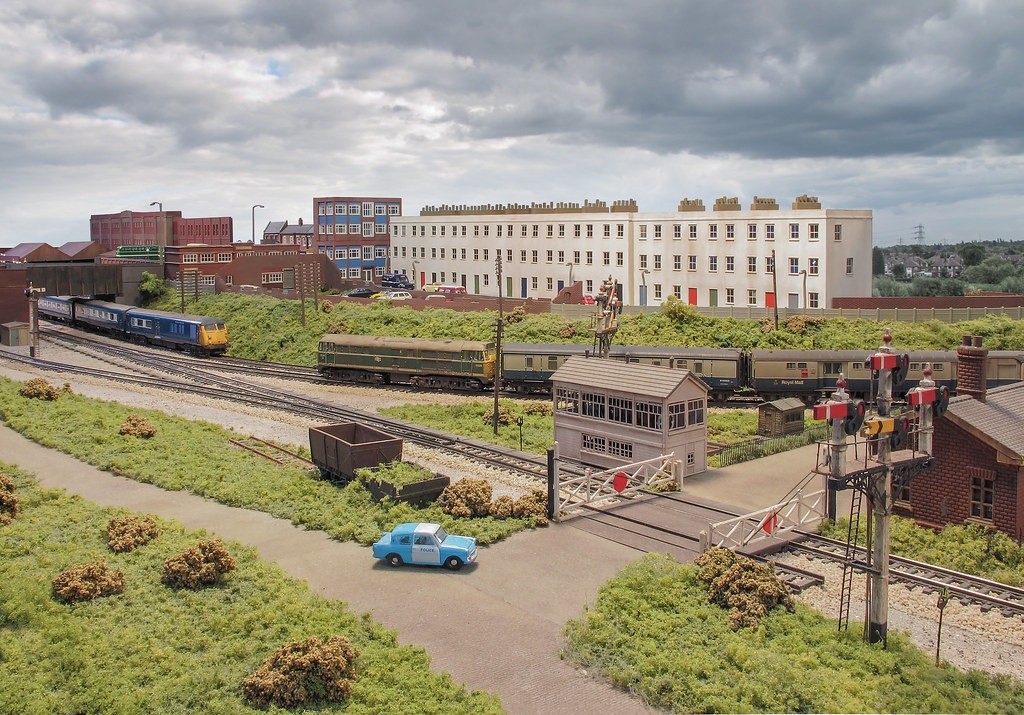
381, 274, 415, 290
434, 285, 468, 295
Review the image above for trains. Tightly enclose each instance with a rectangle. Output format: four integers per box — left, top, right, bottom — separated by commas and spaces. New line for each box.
312, 332, 1024, 403
36, 295, 230, 356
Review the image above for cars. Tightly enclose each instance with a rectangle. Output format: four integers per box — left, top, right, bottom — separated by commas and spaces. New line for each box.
346, 287, 380, 297
371, 522, 478, 571
423, 282, 445, 294
372, 290, 413, 300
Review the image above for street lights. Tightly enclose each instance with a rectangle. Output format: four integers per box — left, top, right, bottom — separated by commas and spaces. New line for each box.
149, 201, 164, 212
798, 269, 808, 311
413, 259, 420, 289
639, 269, 653, 306
565, 262, 575, 287
251, 204, 264, 246
